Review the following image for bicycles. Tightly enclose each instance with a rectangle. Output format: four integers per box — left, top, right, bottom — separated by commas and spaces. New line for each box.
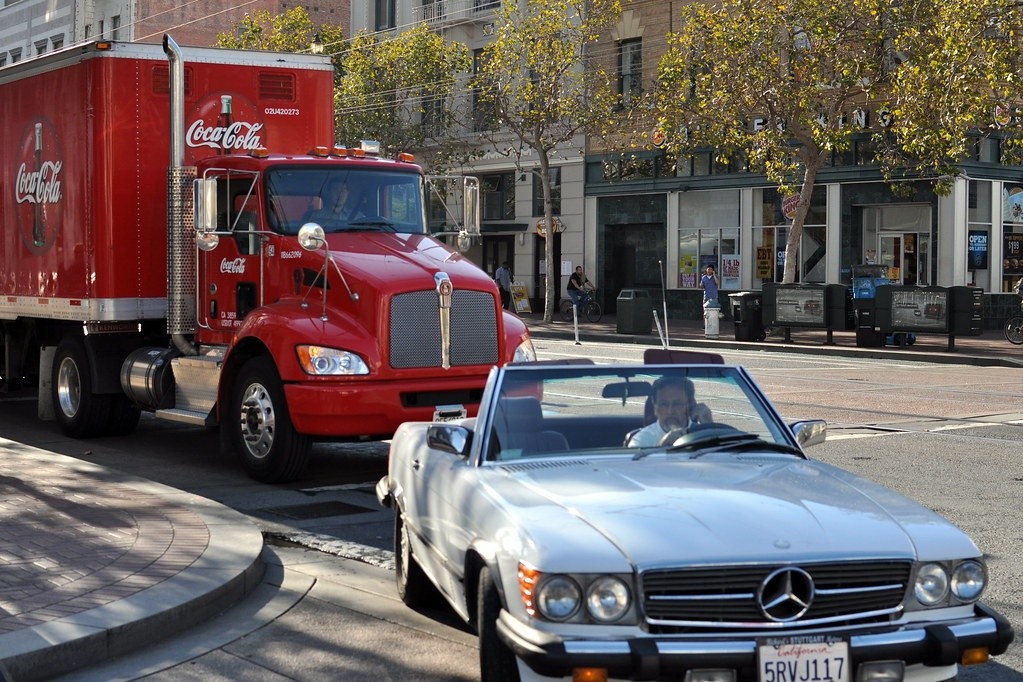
560, 288, 602, 324
1005, 315, 1023, 345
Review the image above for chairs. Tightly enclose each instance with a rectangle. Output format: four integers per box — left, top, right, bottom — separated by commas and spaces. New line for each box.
496, 396, 567, 456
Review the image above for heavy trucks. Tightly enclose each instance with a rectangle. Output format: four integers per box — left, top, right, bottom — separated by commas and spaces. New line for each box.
0, 33, 543, 482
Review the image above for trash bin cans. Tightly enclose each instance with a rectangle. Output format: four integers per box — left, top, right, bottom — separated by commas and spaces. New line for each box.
851, 297, 887, 348
727, 290, 766, 343
616, 288, 652, 336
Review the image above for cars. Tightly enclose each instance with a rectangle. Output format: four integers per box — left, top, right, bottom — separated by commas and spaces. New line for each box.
376, 362, 1014, 682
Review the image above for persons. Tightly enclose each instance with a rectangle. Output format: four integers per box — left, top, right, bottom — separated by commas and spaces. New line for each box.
1014, 275, 1023, 312
496, 262, 513, 309
567, 265, 596, 317
700, 266, 719, 330
628, 377, 712, 449
310, 178, 365, 223
